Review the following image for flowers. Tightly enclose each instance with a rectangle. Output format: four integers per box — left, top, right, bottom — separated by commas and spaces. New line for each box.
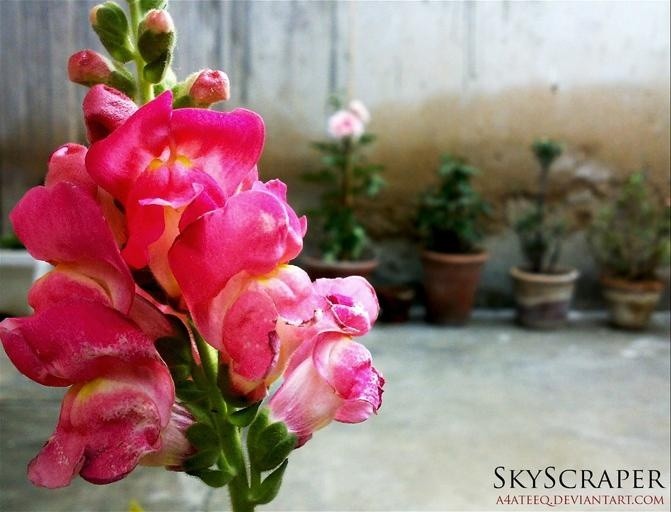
0, 0, 398, 512
297, 91, 393, 263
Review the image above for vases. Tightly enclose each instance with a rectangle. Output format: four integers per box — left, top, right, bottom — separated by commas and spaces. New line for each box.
292, 247, 381, 288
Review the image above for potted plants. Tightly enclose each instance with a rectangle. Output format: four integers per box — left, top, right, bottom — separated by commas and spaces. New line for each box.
407, 149, 508, 334
585, 170, 671, 335
497, 133, 603, 335
